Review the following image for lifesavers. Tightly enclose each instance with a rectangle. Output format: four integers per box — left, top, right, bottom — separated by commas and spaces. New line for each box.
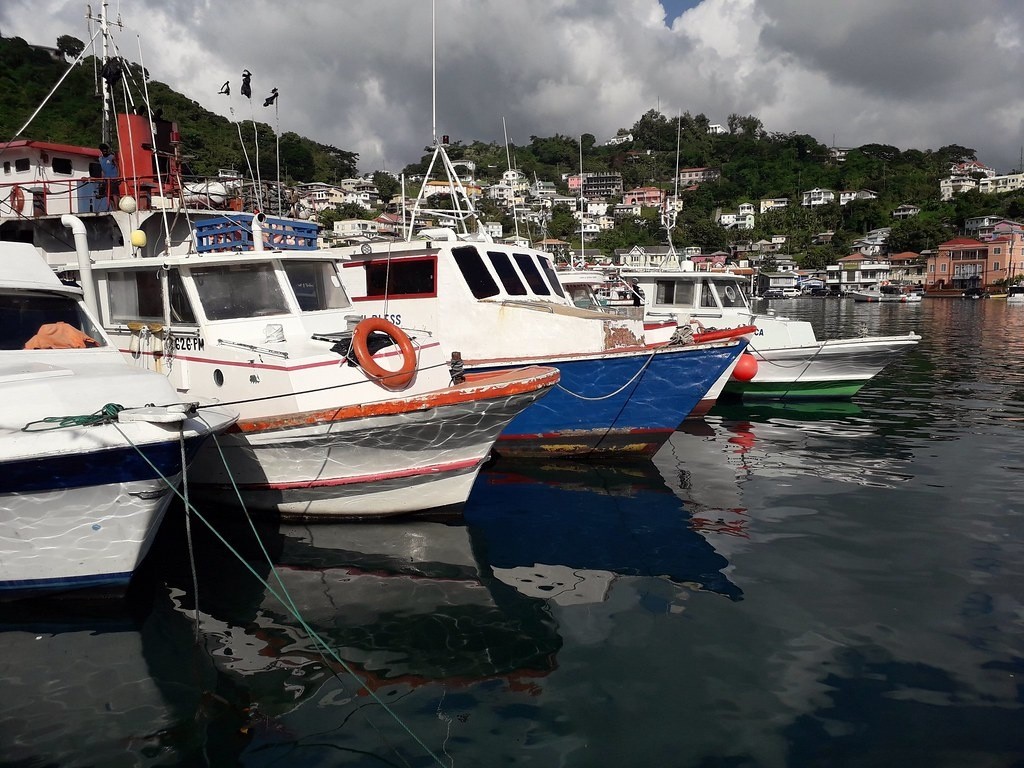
355, 318, 416, 386
10, 186, 24, 211
724, 286, 736, 300
882, 279, 889, 286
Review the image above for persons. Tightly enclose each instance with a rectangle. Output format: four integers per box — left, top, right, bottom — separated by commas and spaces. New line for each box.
99, 144, 120, 210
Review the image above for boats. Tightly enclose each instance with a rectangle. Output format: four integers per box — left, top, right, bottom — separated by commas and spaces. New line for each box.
847, 280, 922, 303
613, 208, 922, 402
552, 246, 758, 422
0, 237, 241, 604
328, 135, 752, 466
0, 1, 562, 519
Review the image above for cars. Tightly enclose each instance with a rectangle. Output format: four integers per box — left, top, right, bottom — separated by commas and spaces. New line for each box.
960, 288, 984, 295
913, 288, 925, 295
762, 288, 843, 297
